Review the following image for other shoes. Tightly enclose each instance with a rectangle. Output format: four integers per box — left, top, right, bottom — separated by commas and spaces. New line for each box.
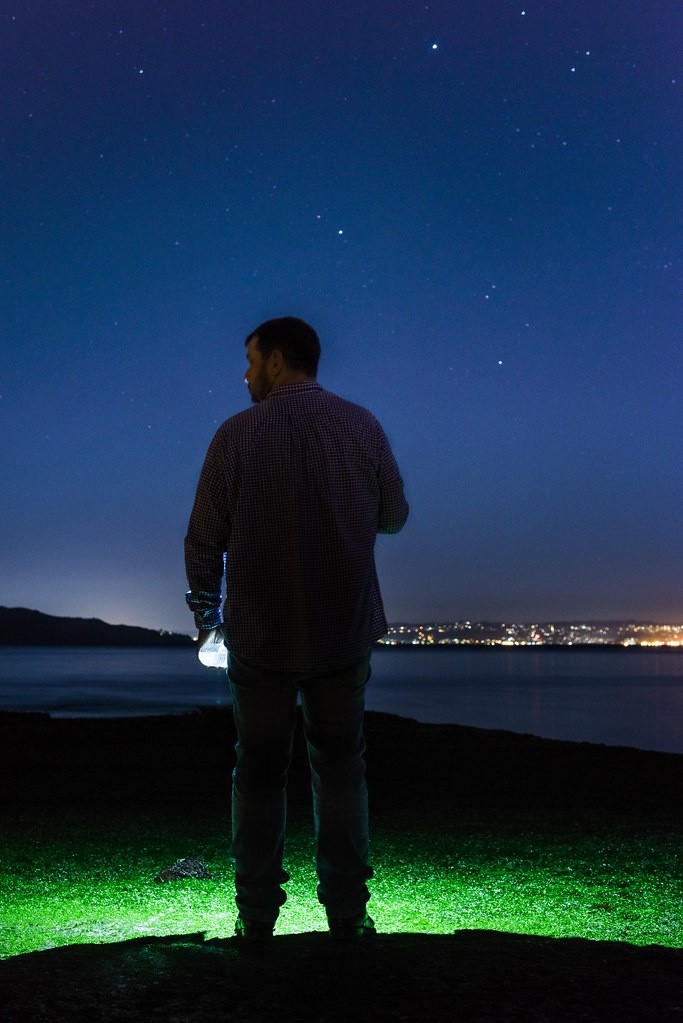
328, 912, 377, 942
235, 916, 275, 939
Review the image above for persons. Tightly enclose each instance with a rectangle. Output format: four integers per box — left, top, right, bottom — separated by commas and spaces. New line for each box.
185, 315, 411, 948
